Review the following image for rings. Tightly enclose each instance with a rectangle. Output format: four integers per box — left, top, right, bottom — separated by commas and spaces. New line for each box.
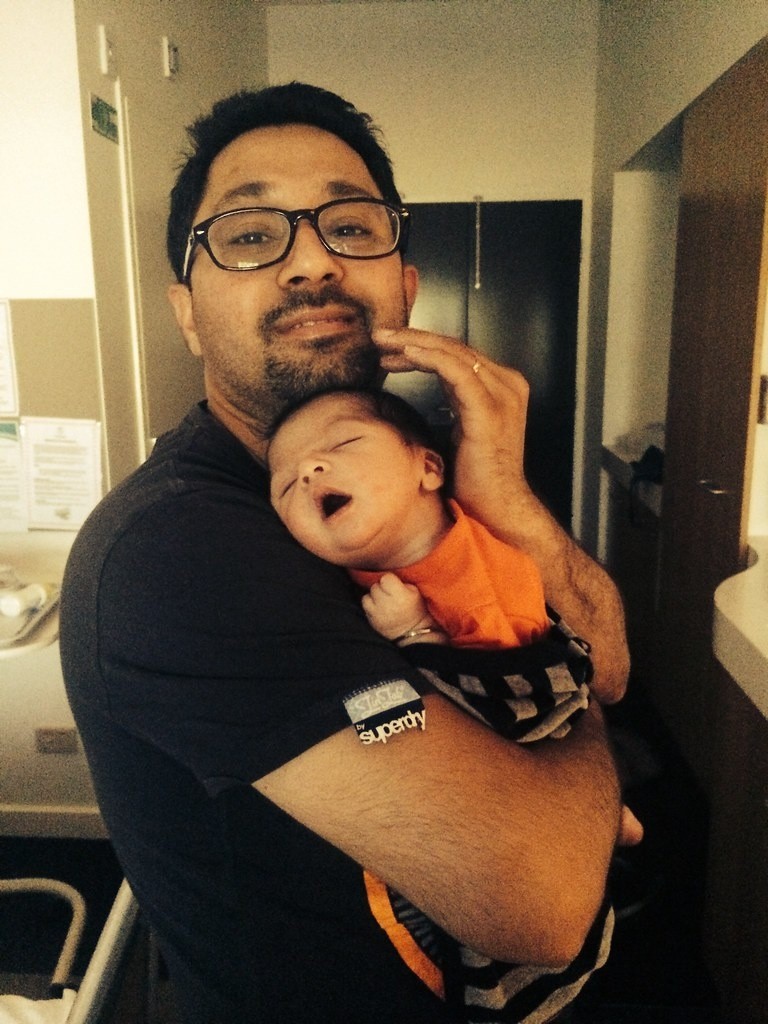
474, 361, 482, 372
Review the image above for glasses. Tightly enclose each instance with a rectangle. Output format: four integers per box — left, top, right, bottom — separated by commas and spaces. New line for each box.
179, 196, 411, 278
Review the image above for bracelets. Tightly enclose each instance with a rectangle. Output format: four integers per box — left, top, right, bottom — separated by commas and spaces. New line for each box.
394, 627, 440, 643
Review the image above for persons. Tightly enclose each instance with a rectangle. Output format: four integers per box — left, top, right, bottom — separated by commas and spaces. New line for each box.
263, 384, 643, 845
59, 81, 651, 1024
